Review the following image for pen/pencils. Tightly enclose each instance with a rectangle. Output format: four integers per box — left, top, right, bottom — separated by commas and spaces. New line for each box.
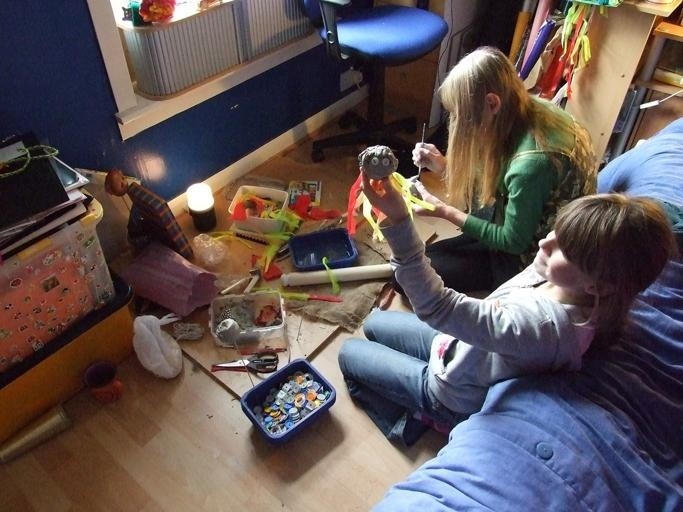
234, 232, 270, 246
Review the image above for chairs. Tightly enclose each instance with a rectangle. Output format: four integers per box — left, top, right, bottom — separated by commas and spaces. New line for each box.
298, 0, 450, 173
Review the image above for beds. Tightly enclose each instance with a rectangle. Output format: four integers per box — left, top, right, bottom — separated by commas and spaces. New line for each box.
381, 118, 683, 511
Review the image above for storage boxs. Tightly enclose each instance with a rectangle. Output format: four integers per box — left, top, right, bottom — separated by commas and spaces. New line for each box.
0, 189, 137, 450
238, 358, 338, 445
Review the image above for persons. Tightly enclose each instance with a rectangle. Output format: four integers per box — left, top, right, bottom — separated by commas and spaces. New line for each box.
292, 182, 319, 206
393, 47, 595, 292
335, 168, 673, 450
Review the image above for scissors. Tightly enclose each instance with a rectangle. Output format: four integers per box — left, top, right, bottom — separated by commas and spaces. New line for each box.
216, 349, 278, 373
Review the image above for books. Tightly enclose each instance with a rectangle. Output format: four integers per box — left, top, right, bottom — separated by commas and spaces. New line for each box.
0, 151, 89, 257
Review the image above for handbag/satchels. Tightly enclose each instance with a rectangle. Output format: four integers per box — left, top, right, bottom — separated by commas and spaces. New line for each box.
0, 131, 70, 230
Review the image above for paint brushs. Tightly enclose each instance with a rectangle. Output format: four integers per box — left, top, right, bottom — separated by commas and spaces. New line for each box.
418, 123, 427, 174
